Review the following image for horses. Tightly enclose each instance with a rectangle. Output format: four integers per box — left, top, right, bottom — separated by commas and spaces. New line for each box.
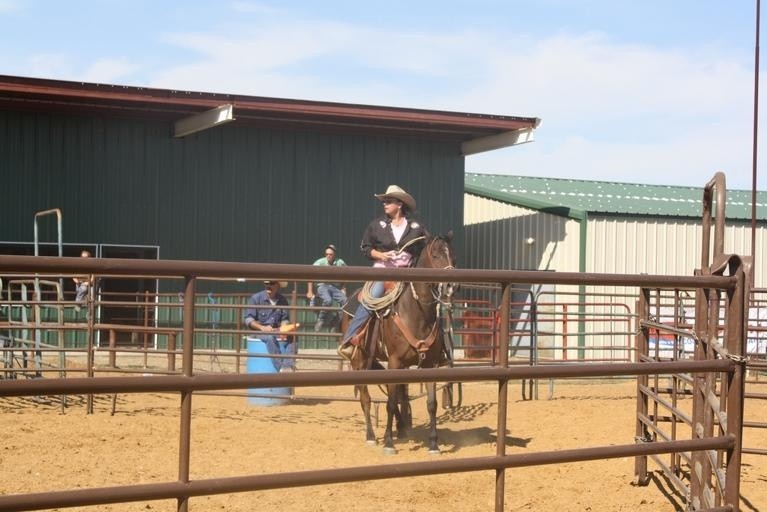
340, 227, 461, 455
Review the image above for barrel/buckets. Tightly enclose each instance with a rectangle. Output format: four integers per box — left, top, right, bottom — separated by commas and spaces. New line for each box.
245, 335, 296, 403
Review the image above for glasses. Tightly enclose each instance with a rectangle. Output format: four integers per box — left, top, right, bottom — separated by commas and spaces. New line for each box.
380, 198, 398, 205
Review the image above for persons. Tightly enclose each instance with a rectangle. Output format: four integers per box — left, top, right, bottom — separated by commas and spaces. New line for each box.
306, 244, 349, 332
72, 249, 94, 320
244, 280, 299, 372
338, 183, 430, 360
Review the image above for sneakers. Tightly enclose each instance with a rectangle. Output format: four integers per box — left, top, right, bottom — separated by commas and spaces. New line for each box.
336, 343, 355, 359
314, 322, 337, 337
279, 365, 297, 373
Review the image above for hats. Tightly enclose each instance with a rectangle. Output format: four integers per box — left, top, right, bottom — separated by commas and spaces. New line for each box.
263, 280, 276, 285
374, 185, 417, 210
